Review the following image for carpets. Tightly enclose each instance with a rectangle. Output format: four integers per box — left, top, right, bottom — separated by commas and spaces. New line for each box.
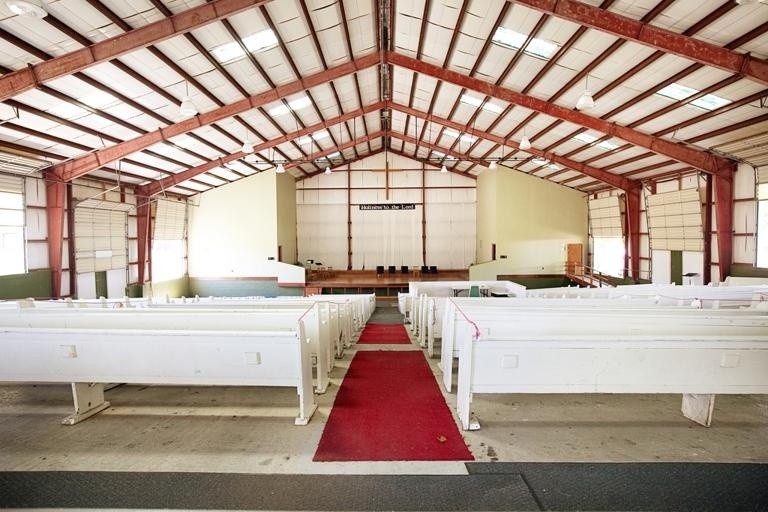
312, 349, 473, 461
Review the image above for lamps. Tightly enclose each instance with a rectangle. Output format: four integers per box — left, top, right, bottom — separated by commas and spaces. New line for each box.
440, 71, 595, 175
179, 80, 332, 176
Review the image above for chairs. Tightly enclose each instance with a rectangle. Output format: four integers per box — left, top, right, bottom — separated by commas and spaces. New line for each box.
376, 265, 438, 279
